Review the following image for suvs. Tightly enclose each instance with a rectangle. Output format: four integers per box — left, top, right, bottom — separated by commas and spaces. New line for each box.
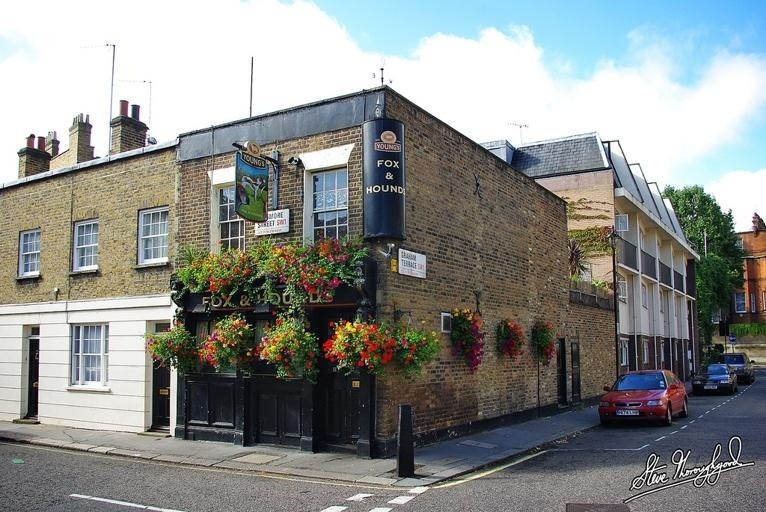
712, 352, 757, 385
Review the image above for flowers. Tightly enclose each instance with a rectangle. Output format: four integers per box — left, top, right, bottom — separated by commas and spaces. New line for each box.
497, 317, 529, 364
450, 307, 487, 375
530, 318, 561, 367
141, 232, 446, 385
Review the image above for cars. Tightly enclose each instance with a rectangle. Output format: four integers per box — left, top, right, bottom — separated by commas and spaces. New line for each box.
596, 369, 689, 426
691, 364, 739, 397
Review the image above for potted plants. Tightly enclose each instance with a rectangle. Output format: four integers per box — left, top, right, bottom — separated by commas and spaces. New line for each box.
568, 272, 606, 298
